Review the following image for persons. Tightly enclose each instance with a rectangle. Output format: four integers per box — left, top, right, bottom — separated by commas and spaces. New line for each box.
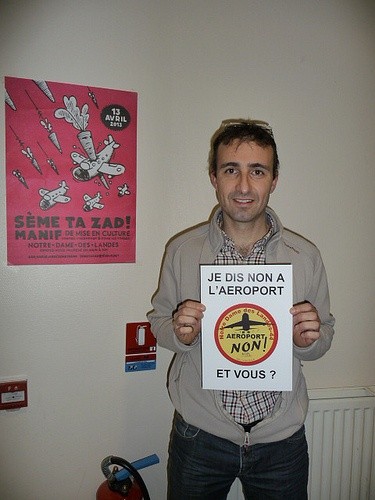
146, 120, 334, 500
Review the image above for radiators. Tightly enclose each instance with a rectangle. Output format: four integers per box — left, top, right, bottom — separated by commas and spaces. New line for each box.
235, 385, 375, 500
